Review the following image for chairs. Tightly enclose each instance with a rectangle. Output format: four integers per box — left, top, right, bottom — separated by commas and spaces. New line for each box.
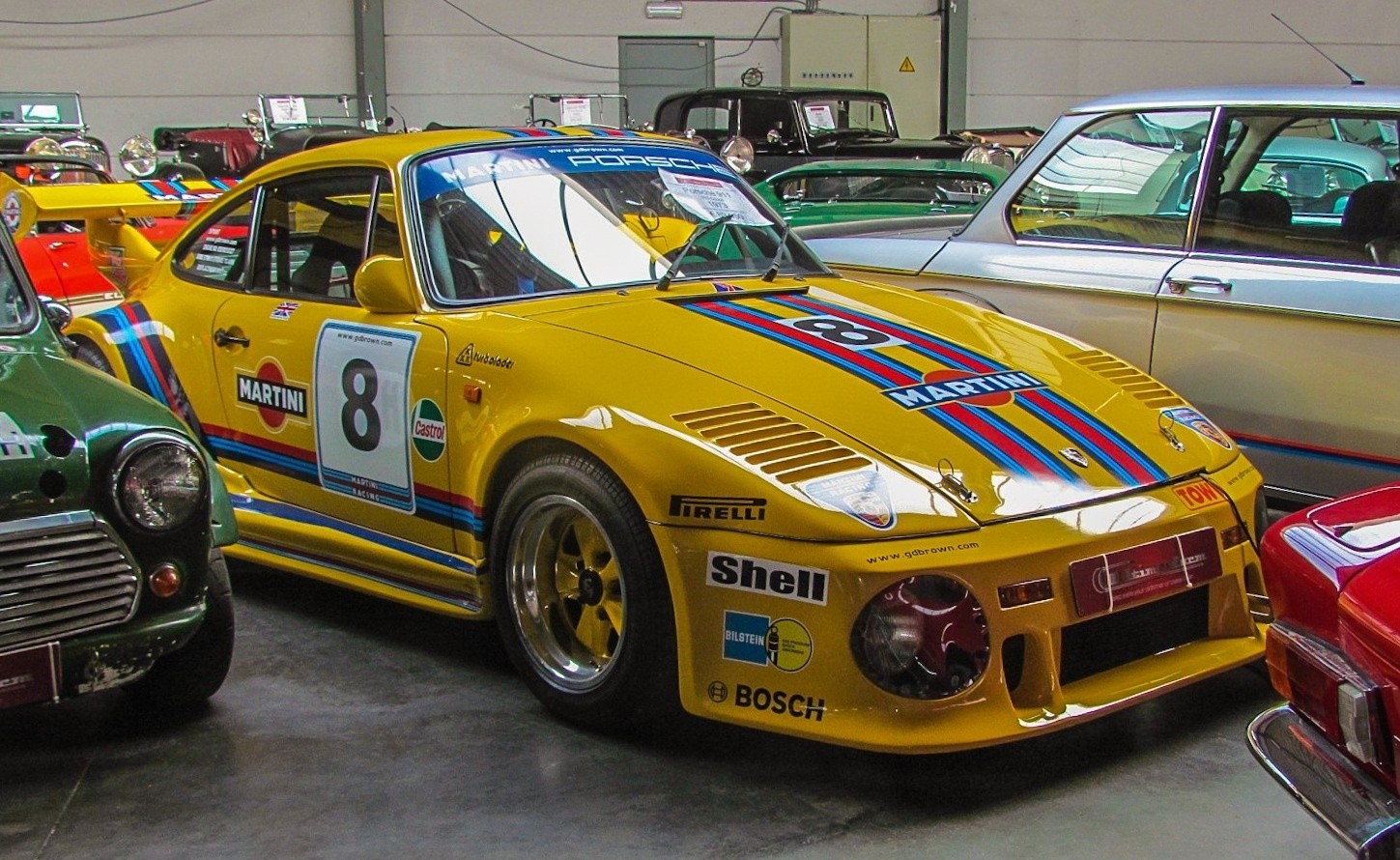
1340, 181, 1399, 241
291, 205, 401, 299
1218, 190, 1291, 229
729, 97, 780, 139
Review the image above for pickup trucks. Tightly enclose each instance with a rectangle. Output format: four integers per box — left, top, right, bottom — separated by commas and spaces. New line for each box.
781, 84, 1400, 511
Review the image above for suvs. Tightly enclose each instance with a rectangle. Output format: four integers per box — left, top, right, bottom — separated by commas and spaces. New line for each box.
653, 86, 1012, 205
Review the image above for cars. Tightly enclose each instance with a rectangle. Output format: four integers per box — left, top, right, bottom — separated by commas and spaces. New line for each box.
1245, 478, 1400, 860
156, 124, 269, 180
949, 126, 1046, 166
1154, 134, 1400, 232
0, 90, 250, 301
0, 124, 1270, 759
680, 159, 1010, 265
0, 205, 238, 741
